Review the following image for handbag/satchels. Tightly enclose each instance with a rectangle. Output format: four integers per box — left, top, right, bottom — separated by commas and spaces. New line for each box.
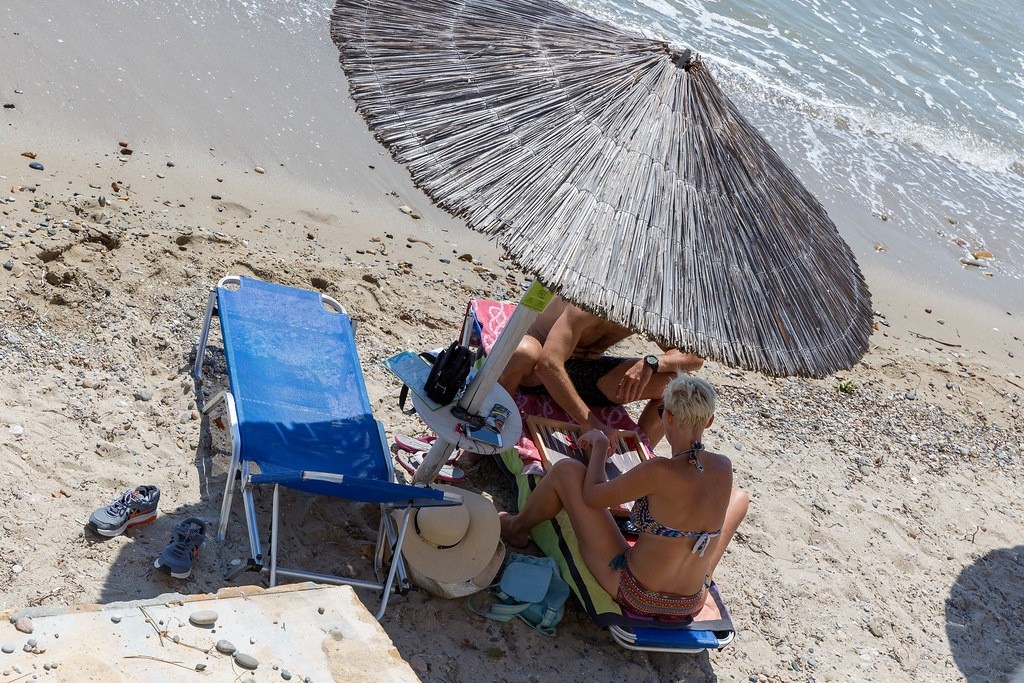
398, 338, 477, 416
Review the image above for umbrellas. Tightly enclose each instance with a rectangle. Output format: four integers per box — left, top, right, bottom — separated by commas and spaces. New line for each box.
331, 0, 874, 487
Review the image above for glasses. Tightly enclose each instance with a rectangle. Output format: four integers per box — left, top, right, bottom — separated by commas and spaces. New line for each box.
657, 404, 674, 420
449, 405, 487, 428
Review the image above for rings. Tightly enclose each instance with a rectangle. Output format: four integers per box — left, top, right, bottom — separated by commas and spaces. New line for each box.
608, 446, 611, 449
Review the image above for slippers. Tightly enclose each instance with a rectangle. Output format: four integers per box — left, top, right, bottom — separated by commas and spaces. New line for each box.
392, 432, 461, 463
395, 448, 466, 485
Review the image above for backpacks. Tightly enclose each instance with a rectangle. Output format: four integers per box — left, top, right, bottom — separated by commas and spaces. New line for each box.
465, 552, 573, 638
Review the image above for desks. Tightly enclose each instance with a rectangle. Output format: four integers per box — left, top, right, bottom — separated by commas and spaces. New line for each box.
412, 366, 522, 496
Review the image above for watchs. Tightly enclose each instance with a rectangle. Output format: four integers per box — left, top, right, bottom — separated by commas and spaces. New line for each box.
644, 355, 658, 373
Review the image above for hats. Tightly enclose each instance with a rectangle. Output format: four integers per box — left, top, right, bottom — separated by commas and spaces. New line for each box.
393, 483, 501, 583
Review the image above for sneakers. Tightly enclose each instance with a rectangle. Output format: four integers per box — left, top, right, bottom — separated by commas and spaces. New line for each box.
154, 516, 206, 579
87, 483, 160, 537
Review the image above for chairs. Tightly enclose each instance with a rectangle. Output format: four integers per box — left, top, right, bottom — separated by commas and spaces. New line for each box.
193, 275, 464, 623
460, 297, 736, 654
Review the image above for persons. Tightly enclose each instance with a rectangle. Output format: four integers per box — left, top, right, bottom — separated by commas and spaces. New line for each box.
499, 292, 706, 458
494, 373, 750, 619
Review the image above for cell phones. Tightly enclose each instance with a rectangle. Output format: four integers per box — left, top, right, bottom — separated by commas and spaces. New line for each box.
466, 424, 503, 448
